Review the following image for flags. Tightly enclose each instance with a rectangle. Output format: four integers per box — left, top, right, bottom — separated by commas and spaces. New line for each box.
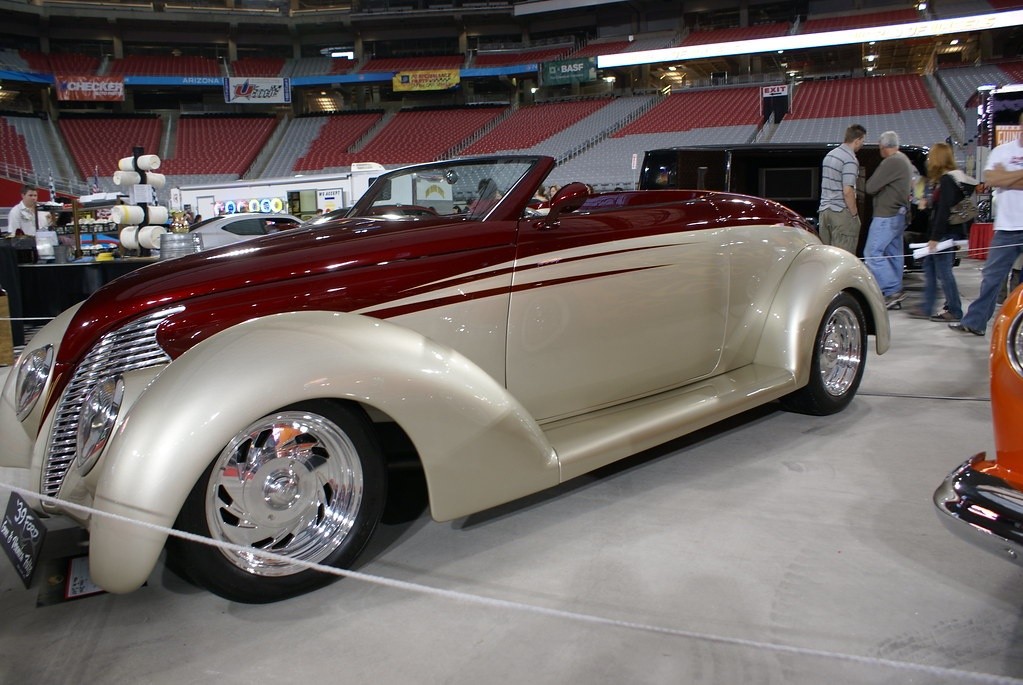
92, 166, 103, 219
48, 169, 57, 201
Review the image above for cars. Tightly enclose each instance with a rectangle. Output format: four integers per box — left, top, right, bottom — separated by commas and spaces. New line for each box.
1, 153, 889, 604
932, 281, 1022, 569
184, 211, 305, 253
270, 206, 440, 235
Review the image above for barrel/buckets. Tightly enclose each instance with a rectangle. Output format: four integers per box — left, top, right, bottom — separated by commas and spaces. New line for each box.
53, 244, 73, 263
158, 233, 205, 263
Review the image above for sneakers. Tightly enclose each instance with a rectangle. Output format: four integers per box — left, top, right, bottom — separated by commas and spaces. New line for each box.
885, 292, 907, 308
932, 311, 961, 322
949, 321, 985, 337
904, 307, 929, 320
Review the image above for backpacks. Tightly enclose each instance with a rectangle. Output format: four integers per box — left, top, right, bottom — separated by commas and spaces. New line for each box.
947, 175, 978, 225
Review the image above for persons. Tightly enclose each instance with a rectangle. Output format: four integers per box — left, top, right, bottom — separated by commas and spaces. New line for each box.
182, 211, 203, 227
9, 184, 55, 238
818, 113, 1023, 336
313, 208, 325, 217
429, 178, 621, 217
